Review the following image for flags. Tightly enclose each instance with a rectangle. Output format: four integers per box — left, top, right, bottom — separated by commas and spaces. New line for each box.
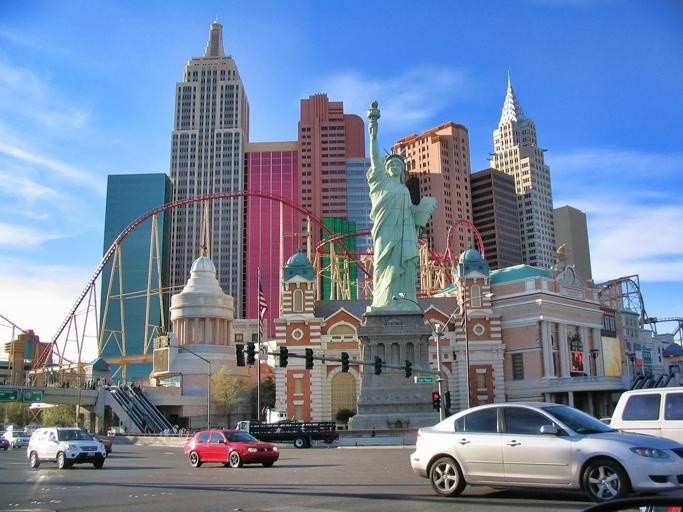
259, 277, 269, 338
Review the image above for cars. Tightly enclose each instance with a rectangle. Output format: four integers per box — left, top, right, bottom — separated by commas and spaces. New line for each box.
406, 400, 682, 503
0, 426, 112, 469
583, 489, 683, 511
184, 429, 279, 470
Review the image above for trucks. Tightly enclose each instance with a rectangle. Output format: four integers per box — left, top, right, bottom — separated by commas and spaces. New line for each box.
236, 419, 339, 448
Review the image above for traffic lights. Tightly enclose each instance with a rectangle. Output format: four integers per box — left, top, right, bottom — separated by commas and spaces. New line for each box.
340, 351, 349, 373
304, 348, 313, 369
374, 356, 381, 375
246, 342, 254, 364
405, 360, 412, 378
279, 346, 288, 368
236, 344, 245, 366
445, 390, 451, 408
432, 392, 440, 409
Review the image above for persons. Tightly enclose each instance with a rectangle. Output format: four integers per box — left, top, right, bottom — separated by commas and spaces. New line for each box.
365, 115, 437, 307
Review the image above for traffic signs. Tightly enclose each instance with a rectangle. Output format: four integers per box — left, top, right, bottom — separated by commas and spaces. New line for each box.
23, 390, 43, 402
0, 388, 18, 402
414, 377, 433, 383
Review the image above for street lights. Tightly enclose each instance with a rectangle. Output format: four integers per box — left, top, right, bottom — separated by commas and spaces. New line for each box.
164, 344, 211, 429
390, 292, 496, 422
590, 349, 598, 376
628, 352, 637, 377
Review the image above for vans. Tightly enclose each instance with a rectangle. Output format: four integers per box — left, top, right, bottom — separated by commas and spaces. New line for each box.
609, 386, 682, 449
